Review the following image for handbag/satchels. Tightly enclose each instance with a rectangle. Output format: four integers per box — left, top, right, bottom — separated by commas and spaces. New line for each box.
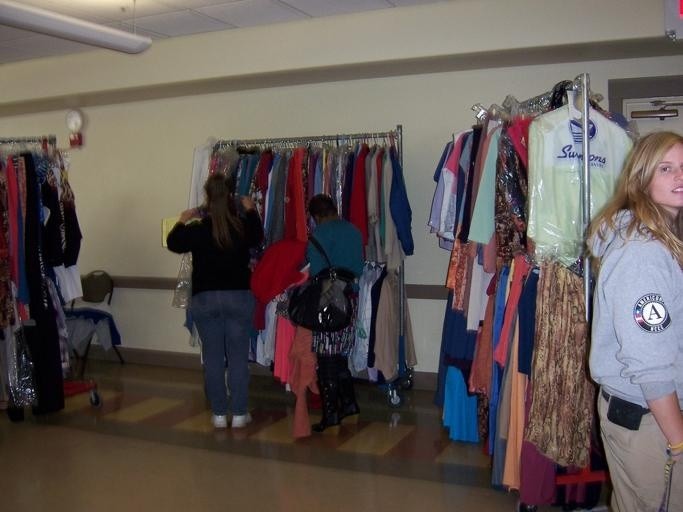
609, 395, 643, 432
289, 234, 354, 332
81, 270, 113, 303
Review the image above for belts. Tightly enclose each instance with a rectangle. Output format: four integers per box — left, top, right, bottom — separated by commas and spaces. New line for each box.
598, 386, 655, 415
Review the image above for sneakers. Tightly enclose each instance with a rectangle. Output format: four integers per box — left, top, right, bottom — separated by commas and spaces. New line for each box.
211, 413, 228, 429
232, 414, 253, 429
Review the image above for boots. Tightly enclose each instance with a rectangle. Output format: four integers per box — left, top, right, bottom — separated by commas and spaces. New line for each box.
337, 356, 361, 417
310, 357, 341, 432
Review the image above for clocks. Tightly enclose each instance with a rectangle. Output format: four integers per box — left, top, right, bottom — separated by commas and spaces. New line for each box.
66, 108, 83, 133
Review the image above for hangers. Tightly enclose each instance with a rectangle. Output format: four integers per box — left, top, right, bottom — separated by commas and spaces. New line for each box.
487, 73, 590, 112
215, 129, 398, 150
0, 136, 62, 157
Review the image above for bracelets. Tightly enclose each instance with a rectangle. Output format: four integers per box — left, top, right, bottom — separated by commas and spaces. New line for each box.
666, 448, 682, 456
666, 440, 683, 448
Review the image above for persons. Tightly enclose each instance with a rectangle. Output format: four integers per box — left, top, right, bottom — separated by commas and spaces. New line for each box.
591, 133, 683, 512
301, 192, 366, 436
166, 174, 268, 430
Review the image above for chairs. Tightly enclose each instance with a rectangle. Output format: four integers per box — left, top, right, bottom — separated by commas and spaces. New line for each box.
66, 271, 124, 364
